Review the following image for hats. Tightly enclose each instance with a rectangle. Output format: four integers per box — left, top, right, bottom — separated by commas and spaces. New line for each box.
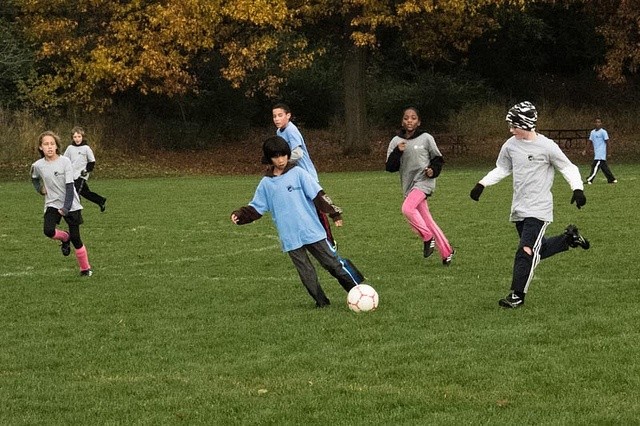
505, 101, 538, 132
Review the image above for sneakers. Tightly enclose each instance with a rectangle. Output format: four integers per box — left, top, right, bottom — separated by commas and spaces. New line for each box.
80, 269, 94, 277
424, 237, 436, 258
315, 303, 328, 309
583, 181, 592, 184
564, 224, 590, 250
58, 231, 70, 256
80, 216, 84, 224
443, 249, 457, 267
333, 240, 339, 251
608, 179, 618, 183
499, 292, 524, 309
100, 198, 106, 212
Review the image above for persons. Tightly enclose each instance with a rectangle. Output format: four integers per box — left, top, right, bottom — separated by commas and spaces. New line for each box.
271, 103, 339, 254
230, 135, 365, 308
63, 125, 107, 212
384, 104, 454, 267
28, 130, 93, 278
469, 99, 590, 312
581, 116, 618, 185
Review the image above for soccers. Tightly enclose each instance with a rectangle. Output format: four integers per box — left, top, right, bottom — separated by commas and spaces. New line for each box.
347, 284, 379, 312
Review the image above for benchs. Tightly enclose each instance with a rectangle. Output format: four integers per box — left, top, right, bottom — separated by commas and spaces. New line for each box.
539, 129, 593, 147
433, 135, 465, 158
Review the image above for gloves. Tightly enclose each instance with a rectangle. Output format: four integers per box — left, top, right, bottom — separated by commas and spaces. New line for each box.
570, 189, 586, 209
469, 182, 485, 201
